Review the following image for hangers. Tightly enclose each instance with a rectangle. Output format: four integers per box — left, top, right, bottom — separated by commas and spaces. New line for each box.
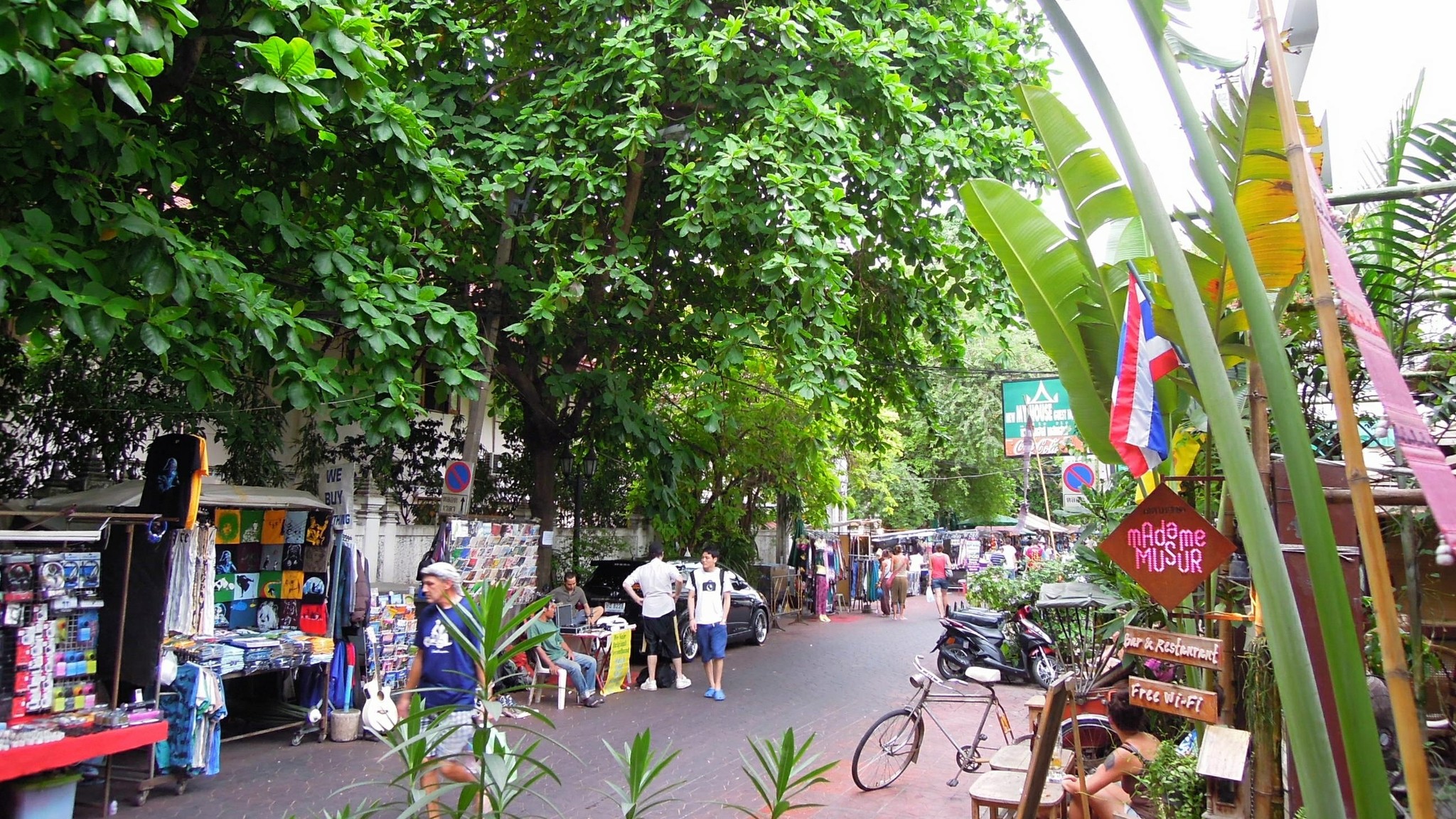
174, 647, 200, 667
333, 530, 352, 547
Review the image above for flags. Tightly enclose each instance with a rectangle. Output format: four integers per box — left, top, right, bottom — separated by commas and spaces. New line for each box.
1108, 273, 1184, 480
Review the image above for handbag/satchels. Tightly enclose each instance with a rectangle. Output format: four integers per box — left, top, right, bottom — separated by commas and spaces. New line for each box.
885, 574, 895, 588
480, 721, 518, 783
926, 586, 935, 602
945, 569, 953, 578
637, 663, 676, 689
876, 587, 883, 599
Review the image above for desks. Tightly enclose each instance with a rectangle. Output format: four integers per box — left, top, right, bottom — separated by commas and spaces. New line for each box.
557, 624, 637, 694
905, 571, 920, 597
967, 744, 1077, 819
172, 661, 332, 767
0, 708, 169, 819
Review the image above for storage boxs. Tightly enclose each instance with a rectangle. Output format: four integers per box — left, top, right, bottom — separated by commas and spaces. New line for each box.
15, 776, 81, 819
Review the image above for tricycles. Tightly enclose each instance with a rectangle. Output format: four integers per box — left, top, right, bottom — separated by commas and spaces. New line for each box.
851, 656, 1122, 791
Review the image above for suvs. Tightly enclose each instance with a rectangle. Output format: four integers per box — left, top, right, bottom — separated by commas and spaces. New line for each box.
575, 557, 773, 663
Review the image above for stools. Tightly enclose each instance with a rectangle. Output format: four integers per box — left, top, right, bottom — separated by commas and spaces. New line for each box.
1026, 694, 1052, 734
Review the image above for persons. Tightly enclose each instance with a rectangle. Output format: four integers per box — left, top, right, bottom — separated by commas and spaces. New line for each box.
876, 534, 1095, 621
398, 561, 495, 819
529, 540, 734, 709
796, 536, 832, 622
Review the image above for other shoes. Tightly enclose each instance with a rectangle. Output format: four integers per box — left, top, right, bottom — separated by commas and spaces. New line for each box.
900, 618, 908, 620
474, 793, 494, 815
881, 614, 891, 617
940, 614, 944, 618
891, 609, 899, 614
704, 688, 714, 697
894, 617, 897, 620
944, 612, 946, 616
715, 690, 725, 700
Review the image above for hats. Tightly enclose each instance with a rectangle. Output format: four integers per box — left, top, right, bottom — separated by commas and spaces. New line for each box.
421, 561, 465, 597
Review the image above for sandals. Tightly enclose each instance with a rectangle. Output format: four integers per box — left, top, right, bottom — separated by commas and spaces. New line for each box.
583, 695, 599, 707
591, 693, 605, 703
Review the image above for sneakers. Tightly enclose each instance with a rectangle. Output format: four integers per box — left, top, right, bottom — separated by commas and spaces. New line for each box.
676, 675, 692, 689
640, 677, 657, 691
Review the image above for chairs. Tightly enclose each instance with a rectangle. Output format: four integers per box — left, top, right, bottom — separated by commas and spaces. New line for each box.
528, 642, 582, 711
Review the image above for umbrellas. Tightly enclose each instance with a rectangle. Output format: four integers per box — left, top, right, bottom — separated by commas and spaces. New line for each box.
958, 512, 1020, 532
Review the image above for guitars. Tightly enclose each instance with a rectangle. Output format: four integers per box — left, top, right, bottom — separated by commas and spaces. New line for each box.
361, 625, 399, 734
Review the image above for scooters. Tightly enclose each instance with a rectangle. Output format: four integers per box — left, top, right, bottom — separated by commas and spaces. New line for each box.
930, 600, 1064, 690
